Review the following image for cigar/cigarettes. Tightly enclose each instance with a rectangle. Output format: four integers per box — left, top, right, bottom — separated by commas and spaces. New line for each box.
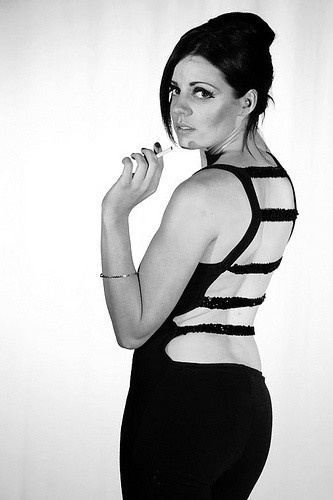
155, 146, 174, 160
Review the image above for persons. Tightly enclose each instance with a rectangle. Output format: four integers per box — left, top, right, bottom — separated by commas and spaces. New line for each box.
101, 12, 298, 500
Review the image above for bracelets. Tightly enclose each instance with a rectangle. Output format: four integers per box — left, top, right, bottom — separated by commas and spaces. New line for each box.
101, 271, 137, 279
